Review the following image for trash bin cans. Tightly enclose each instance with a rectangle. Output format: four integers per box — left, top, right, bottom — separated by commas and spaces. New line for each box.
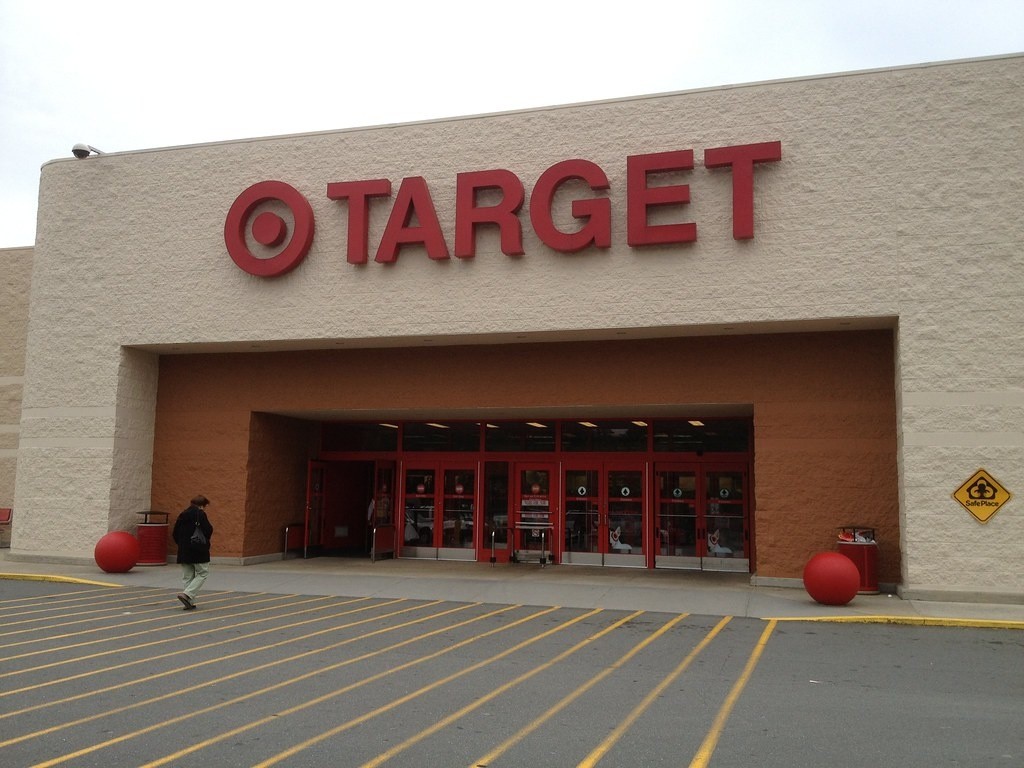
836, 525, 880, 595
135, 511, 171, 566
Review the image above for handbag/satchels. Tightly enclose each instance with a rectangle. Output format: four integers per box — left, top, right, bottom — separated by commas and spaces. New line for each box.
191, 509, 209, 551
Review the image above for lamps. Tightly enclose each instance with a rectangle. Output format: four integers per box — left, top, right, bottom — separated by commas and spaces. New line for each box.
69, 143, 105, 158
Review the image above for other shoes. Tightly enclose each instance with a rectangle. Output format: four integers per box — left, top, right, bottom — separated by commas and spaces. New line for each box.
177, 594, 192, 609
183, 605, 196, 610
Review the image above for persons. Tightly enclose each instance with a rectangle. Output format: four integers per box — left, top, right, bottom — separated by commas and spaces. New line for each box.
172, 494, 213, 610
